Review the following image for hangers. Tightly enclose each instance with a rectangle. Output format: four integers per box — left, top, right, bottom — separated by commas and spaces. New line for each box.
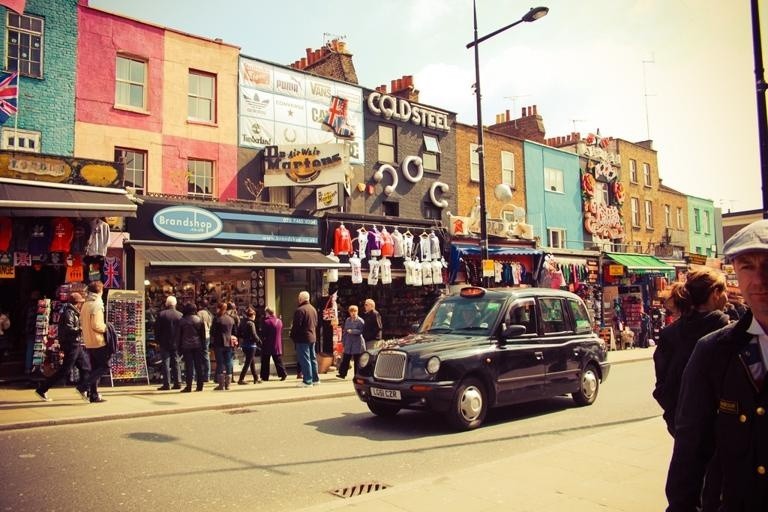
92, 215, 99, 222
337, 220, 438, 238
492, 259, 522, 265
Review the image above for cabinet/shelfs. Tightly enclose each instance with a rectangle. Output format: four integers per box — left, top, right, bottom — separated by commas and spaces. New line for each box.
202, 267, 254, 318
147, 267, 206, 312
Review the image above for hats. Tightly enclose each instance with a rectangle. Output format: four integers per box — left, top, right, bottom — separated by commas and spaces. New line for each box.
719, 218, 768, 261
67, 293, 86, 304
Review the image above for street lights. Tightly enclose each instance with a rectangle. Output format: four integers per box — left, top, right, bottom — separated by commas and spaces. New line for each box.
464, 1, 550, 286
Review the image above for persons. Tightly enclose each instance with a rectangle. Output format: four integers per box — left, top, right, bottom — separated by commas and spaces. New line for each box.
362, 299, 382, 351
0, 286, 58, 374
325, 220, 443, 286
146, 291, 318, 393
455, 306, 483, 328
78, 281, 110, 402
510, 307, 530, 333
35, 292, 92, 400
723, 293, 747, 320
638, 311, 651, 346
652, 217, 766, 512
336, 305, 367, 379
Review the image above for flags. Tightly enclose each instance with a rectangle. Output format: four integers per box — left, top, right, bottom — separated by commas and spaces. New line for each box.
0, 71, 17, 126
103, 259, 120, 289
323, 96, 348, 133
321, 307, 336, 320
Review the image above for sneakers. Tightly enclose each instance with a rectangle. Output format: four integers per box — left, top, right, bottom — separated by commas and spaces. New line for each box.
171, 385, 182, 389
237, 371, 321, 388
213, 384, 232, 390
336, 374, 347, 380
180, 387, 191, 392
75, 384, 107, 404
193, 386, 203, 392
34, 388, 53, 402
157, 384, 171, 391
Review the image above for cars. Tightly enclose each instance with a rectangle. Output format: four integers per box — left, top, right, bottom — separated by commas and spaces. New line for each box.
352, 287, 613, 429
